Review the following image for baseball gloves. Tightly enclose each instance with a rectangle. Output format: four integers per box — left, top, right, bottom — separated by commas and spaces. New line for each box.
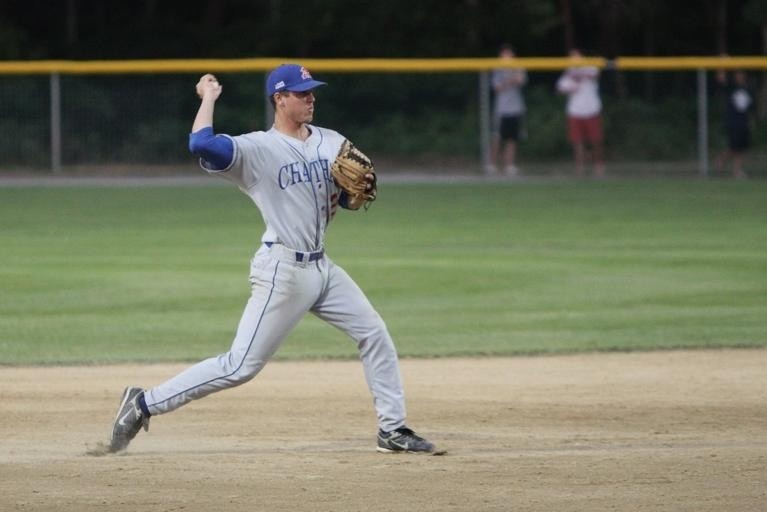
330, 140, 377, 210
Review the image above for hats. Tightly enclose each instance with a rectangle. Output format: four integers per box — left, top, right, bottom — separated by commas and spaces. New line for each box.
266, 64, 329, 97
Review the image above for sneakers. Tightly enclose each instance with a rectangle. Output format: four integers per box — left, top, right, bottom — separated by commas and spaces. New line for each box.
376, 425, 445, 455
108, 385, 149, 456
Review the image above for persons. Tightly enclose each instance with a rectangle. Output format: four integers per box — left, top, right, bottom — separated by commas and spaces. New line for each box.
713, 53, 758, 178
487, 41, 529, 176
550, 47, 618, 172
105, 64, 440, 455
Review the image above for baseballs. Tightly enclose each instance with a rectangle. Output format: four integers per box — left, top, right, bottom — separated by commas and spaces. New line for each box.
211, 80, 219, 89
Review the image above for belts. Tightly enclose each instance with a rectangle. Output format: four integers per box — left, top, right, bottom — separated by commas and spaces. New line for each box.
269, 240, 328, 262
